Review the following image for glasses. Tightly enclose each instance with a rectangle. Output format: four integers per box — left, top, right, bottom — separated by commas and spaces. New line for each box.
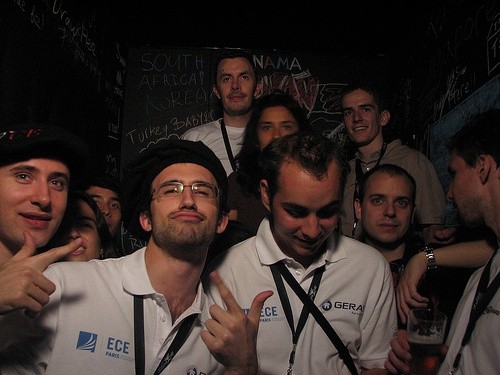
150, 180, 223, 201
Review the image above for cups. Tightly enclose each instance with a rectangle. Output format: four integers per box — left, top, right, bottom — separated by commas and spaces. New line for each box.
406, 305, 447, 375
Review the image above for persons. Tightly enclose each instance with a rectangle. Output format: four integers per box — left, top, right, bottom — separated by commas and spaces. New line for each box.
352, 163, 496, 335
162, 52, 455, 267
0, 125, 124, 318
434, 134, 500, 375
202, 128, 400, 373
2, 161, 274, 375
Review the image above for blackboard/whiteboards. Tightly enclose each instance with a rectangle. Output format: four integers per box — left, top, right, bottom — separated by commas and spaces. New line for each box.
0, 0, 500, 259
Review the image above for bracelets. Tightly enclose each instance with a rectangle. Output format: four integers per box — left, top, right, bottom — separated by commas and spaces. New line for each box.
423, 246, 438, 275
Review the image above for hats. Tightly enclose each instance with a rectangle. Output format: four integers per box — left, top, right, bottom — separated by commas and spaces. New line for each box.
0, 122, 90, 166
119, 140, 229, 242
